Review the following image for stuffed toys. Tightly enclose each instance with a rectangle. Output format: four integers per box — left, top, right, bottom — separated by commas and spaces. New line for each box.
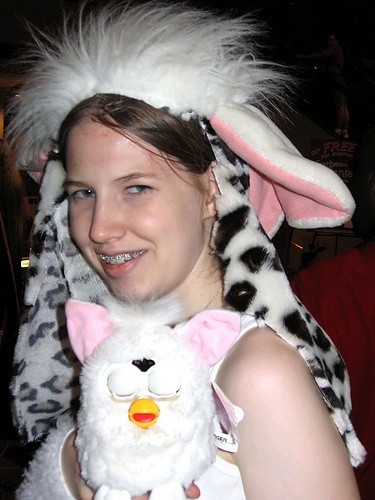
63, 297, 242, 500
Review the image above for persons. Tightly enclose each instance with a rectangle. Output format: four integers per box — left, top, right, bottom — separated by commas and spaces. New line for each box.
0, 92, 361, 499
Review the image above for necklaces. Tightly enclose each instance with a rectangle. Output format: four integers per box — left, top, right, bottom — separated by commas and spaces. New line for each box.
185, 283, 223, 319
